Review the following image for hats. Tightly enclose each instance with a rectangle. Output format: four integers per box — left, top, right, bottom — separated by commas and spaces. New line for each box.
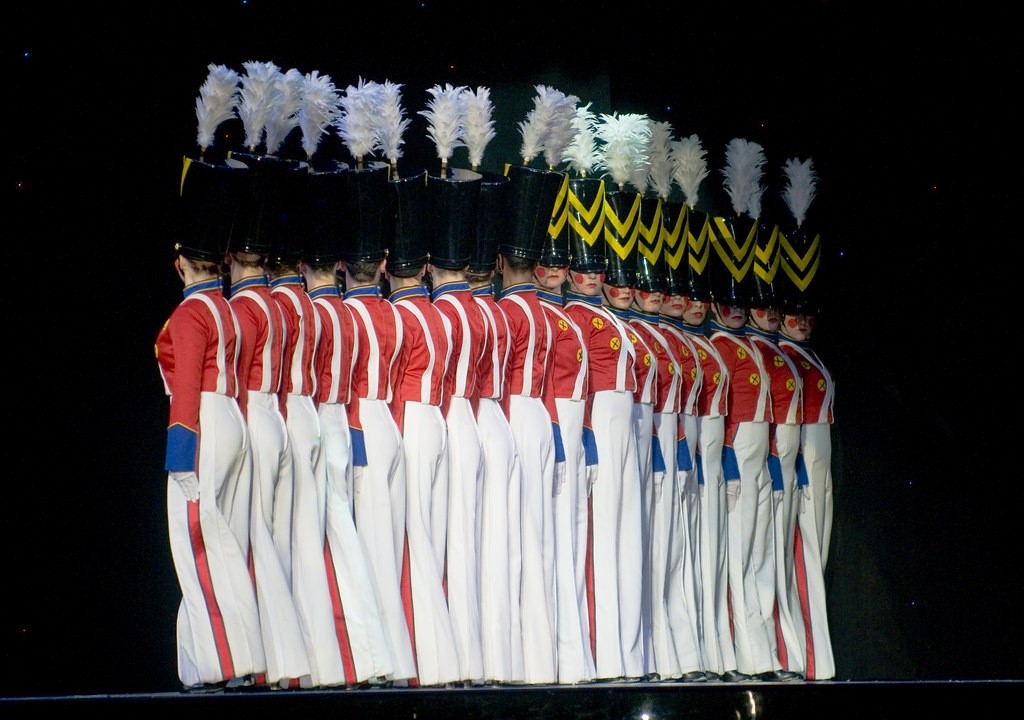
779, 156, 823, 317
169, 61, 785, 315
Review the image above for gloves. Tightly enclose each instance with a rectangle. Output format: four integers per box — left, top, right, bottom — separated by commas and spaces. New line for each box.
797, 483, 811, 515
552, 461, 567, 498
726, 479, 741, 513
698, 483, 704, 499
773, 489, 783, 517
650, 471, 664, 503
168, 471, 201, 504
353, 465, 363, 499
679, 469, 691, 497
586, 463, 598, 498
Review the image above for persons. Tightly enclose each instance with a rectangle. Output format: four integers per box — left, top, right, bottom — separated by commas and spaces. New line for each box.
154, 154, 835, 692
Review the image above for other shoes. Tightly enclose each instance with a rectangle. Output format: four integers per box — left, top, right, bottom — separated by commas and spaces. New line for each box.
177, 671, 833, 693
